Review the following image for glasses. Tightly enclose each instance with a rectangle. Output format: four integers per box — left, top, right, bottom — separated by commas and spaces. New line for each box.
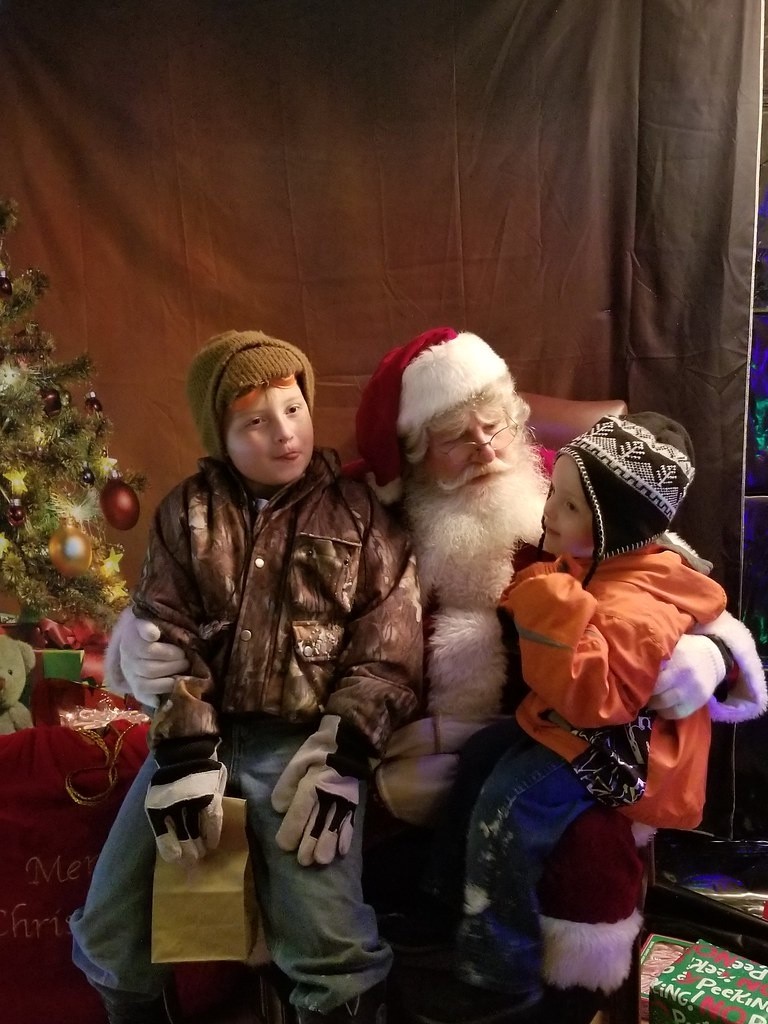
428, 416, 519, 466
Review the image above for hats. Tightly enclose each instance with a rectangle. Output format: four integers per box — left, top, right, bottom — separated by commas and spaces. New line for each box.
536, 410, 697, 589
356, 326, 509, 505
187, 330, 316, 461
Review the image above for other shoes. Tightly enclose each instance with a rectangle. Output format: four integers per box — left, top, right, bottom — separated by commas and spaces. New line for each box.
106, 993, 184, 1024
298, 988, 385, 1024
417, 984, 543, 1024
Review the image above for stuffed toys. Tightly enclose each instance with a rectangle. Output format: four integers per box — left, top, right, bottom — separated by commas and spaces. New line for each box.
0, 635, 36, 735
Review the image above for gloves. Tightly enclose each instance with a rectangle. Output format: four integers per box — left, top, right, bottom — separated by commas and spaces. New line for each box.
270, 712, 361, 867
119, 617, 191, 710
143, 733, 228, 861
646, 630, 727, 721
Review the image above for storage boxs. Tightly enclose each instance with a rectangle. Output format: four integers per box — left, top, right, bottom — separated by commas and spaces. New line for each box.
639, 931, 768, 1024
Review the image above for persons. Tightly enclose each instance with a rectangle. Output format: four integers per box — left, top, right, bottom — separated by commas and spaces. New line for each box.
70, 329, 423, 1024
411, 411, 727, 1024
105, 327, 768, 1024
